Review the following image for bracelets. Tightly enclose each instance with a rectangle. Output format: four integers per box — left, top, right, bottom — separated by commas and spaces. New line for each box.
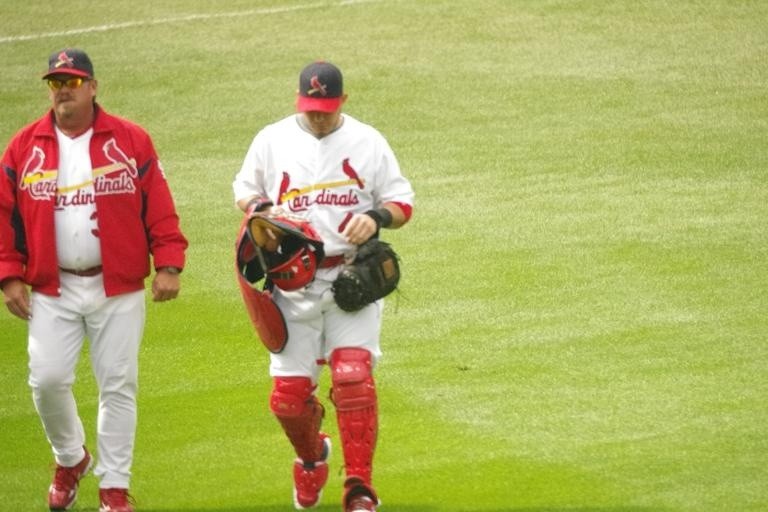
370, 205, 392, 226
247, 196, 271, 212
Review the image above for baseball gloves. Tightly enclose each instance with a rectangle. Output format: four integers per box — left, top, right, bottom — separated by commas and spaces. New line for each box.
331, 240, 400, 311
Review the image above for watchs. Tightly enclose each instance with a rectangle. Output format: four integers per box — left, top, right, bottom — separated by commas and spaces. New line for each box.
159, 265, 181, 276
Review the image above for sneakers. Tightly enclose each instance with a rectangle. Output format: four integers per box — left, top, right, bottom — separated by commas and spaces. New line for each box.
48, 445, 94, 511
294, 434, 331, 511
343, 493, 378, 511
99, 489, 136, 512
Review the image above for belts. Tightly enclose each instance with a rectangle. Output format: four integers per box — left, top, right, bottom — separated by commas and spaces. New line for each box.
319, 254, 343, 269
59, 265, 101, 276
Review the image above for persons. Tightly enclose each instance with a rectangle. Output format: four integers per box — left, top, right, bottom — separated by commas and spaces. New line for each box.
0, 49, 186, 511
230, 58, 416, 511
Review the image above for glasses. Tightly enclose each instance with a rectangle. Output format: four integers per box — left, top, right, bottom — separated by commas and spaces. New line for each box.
47, 76, 92, 92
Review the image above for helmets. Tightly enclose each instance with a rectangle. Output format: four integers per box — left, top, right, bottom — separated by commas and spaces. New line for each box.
266, 242, 316, 290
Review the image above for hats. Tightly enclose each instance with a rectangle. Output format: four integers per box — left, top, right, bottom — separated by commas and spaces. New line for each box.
297, 62, 342, 113
42, 49, 94, 80
